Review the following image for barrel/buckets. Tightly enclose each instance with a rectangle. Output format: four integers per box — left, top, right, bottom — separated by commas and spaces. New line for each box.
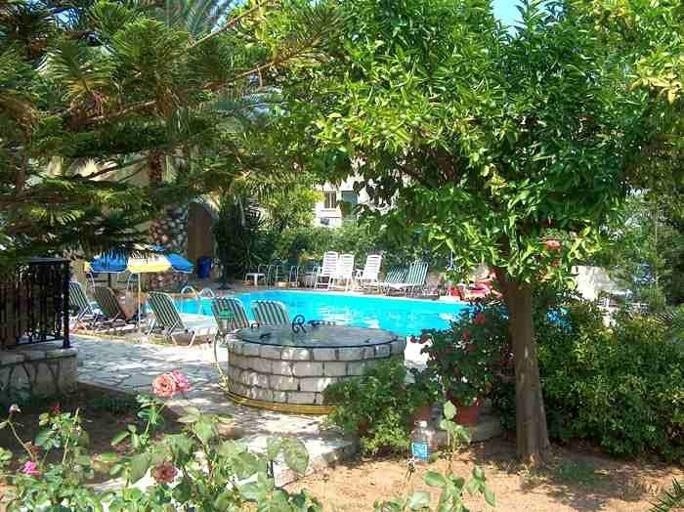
410, 419, 437, 466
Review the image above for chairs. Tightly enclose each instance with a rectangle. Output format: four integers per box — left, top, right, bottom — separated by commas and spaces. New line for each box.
253, 244, 428, 299
66, 279, 291, 348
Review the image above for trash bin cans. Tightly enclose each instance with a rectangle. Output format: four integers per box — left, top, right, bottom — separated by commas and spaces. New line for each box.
197, 256, 211, 278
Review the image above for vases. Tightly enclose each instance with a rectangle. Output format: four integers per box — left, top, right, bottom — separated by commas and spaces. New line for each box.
443, 389, 482, 427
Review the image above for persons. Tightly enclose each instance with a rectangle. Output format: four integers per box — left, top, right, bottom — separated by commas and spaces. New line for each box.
112, 288, 147, 324
72, 250, 90, 297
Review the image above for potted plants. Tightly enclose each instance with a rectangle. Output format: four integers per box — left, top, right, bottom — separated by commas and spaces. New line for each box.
308, 357, 441, 452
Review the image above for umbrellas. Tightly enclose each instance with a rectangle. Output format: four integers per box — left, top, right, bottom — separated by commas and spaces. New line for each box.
91, 241, 196, 331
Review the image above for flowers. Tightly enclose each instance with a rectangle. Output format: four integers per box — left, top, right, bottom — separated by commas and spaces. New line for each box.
410, 308, 509, 427
